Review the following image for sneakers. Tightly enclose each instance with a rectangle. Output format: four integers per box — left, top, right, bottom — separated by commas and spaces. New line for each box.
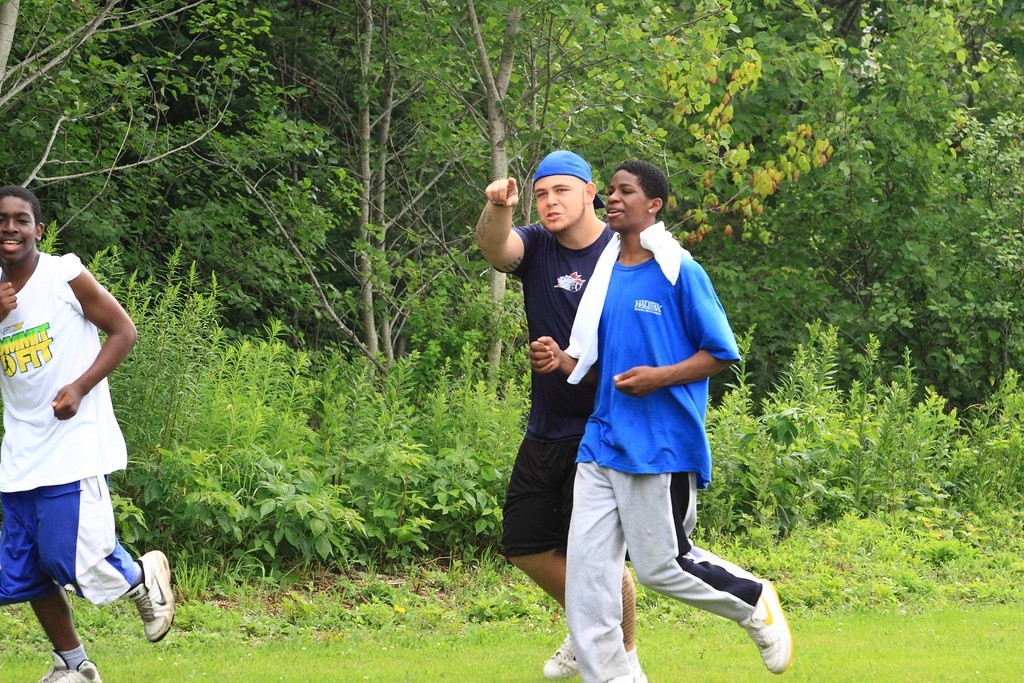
543, 632, 577, 681
120, 550, 176, 642
36, 651, 103, 683
606, 674, 639, 683
628, 647, 647, 683
738, 578, 793, 674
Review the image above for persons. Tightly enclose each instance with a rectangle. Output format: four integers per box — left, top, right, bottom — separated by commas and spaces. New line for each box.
474, 149, 647, 683
0, 185, 175, 683
529, 158, 792, 682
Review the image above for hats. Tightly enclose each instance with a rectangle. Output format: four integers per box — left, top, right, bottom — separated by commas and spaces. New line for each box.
533, 150, 606, 210
